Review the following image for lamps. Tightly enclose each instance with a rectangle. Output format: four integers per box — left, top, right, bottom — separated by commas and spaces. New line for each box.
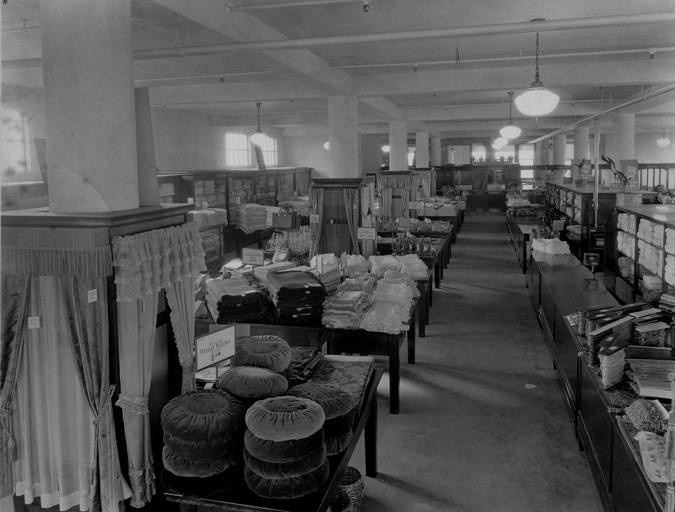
497, 18, 561, 139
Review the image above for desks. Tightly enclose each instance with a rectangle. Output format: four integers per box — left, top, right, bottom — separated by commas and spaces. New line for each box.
194, 264, 434, 415
160, 354, 386, 511
375, 197, 464, 303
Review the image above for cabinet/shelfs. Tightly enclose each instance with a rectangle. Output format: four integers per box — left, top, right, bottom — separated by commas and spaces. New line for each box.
545, 180, 675, 306
181, 166, 313, 255
